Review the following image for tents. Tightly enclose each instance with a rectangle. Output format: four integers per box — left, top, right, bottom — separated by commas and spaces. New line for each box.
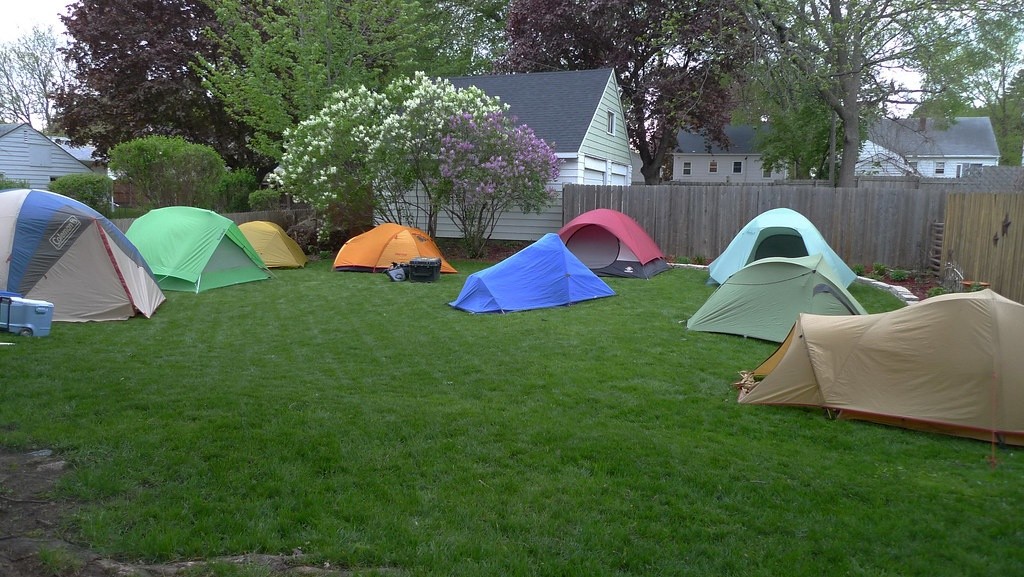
448, 232, 618, 315
703, 207, 858, 290
0, 188, 167, 323
557, 208, 673, 281
685, 256, 870, 343
124, 206, 278, 295
737, 288, 1024, 447
331, 222, 459, 273
237, 220, 309, 268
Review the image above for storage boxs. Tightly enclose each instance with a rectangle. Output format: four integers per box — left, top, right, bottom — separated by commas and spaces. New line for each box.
409, 257, 440, 281
